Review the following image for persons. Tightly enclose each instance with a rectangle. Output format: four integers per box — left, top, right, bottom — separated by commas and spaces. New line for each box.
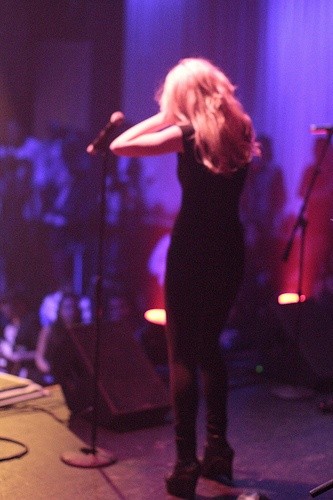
1, 114, 168, 387
238, 134, 333, 304
109, 55, 260, 498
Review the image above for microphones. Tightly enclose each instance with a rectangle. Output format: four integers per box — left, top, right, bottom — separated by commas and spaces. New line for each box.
311, 125, 333, 135
86, 110, 123, 155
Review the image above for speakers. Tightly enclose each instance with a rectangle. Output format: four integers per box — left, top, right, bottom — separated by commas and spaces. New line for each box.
44, 323, 171, 431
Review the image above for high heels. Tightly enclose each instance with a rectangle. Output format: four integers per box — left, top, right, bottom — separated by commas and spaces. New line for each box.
200, 443, 234, 485
167, 459, 202, 500
